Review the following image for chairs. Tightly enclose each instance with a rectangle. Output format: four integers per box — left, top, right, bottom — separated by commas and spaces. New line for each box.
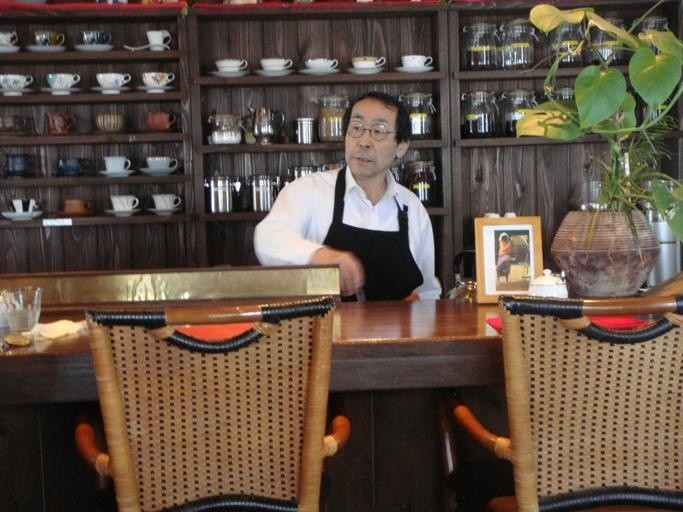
442, 278, 681, 511
76, 296, 351, 512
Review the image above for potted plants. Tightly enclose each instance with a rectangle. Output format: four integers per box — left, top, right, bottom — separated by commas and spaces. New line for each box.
516, 3, 682, 298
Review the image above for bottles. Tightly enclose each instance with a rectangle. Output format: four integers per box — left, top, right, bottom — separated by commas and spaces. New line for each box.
319, 95, 349, 141
208, 113, 242, 145
461, 16, 677, 138
403, 161, 436, 206
401, 93, 434, 139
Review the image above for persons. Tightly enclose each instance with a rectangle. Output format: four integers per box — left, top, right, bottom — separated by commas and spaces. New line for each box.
252, 91, 442, 301
496, 232, 512, 282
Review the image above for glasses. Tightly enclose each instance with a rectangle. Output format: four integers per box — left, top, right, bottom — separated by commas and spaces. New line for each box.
348, 120, 396, 141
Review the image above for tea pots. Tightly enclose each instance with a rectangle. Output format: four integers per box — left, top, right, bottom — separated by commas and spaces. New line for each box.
247, 104, 285, 147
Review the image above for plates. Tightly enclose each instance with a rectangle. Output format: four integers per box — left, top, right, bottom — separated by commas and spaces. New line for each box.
212, 67, 434, 77
0, 44, 179, 221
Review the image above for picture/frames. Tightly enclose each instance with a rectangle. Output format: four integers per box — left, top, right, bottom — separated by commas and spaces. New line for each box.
476, 211, 545, 306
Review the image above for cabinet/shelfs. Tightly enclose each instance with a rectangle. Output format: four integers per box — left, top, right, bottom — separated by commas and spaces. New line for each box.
190, 1, 442, 294
0, 1, 180, 264
447, 4, 683, 290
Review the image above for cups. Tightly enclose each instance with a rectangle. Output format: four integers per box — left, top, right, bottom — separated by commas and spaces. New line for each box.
0, 31, 183, 212
1, 286, 42, 337
215, 55, 433, 72
294, 118, 315, 144
579, 181, 605, 212
528, 268, 568, 298
